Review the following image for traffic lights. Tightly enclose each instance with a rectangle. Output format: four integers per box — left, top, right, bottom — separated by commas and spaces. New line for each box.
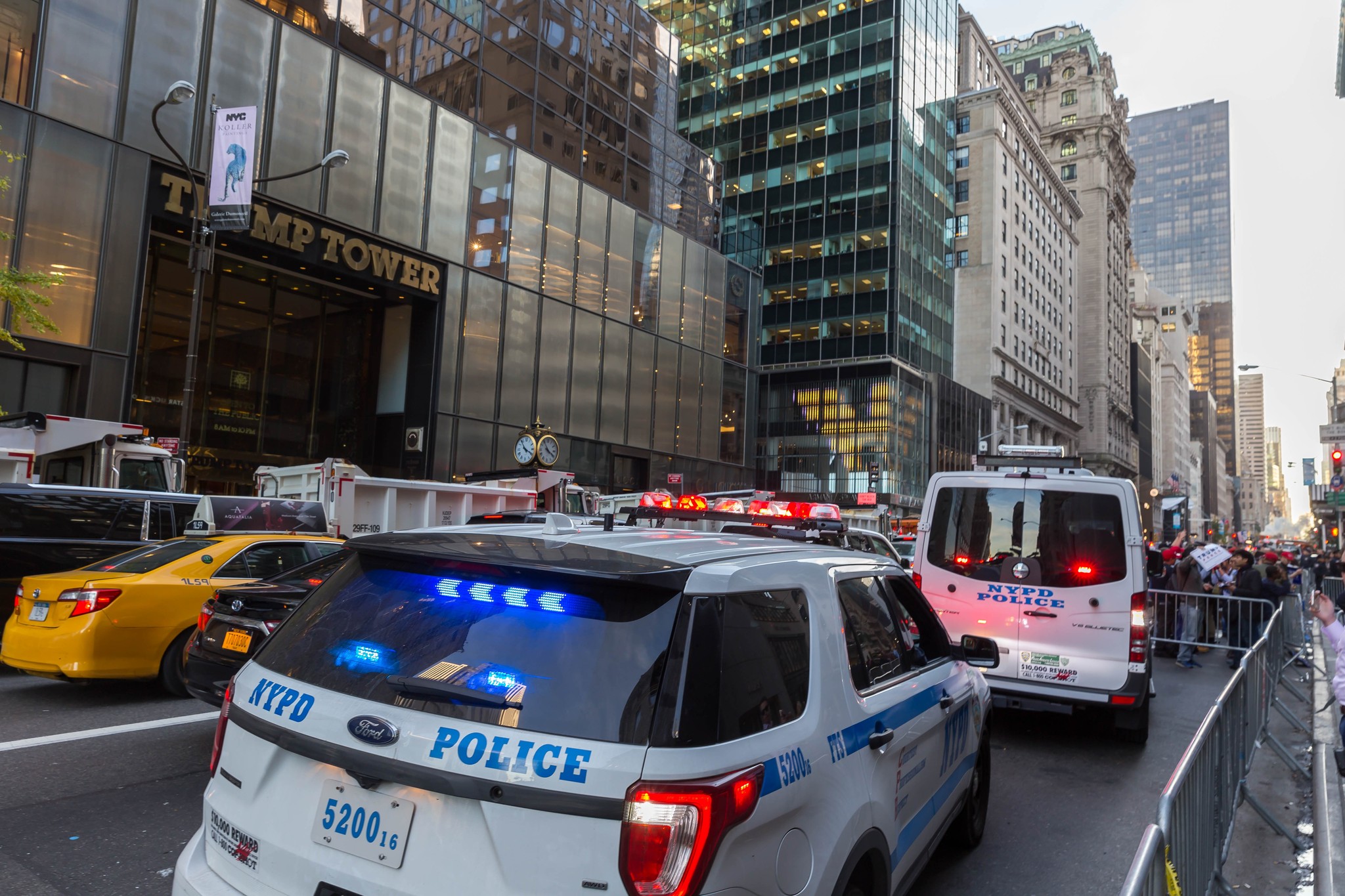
1331, 450, 1343, 473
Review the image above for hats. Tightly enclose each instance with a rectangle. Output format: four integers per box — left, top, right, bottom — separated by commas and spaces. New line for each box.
1163, 549, 1182, 559
1305, 547, 1311, 553
1281, 551, 1295, 562
1170, 545, 1184, 553
1263, 551, 1278, 563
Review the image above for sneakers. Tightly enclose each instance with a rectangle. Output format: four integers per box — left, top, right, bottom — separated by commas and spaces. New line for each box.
1189, 660, 1202, 668
1176, 661, 1192, 669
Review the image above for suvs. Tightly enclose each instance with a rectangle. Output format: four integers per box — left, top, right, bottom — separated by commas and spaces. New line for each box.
171, 512, 1006, 895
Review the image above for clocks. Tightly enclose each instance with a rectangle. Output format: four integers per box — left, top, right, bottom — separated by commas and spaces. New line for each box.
537, 435, 560, 466
513, 433, 537, 465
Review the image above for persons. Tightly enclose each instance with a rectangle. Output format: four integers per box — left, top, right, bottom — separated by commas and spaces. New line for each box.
1142, 531, 1345, 775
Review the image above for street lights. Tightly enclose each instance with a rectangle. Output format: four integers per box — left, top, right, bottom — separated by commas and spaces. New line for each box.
978, 408, 1029, 453
1237, 364, 1338, 549
152, 81, 348, 474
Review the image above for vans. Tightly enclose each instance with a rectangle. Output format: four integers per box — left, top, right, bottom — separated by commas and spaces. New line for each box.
911, 446, 1156, 739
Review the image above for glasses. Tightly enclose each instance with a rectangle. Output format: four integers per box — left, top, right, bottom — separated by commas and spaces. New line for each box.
1335, 558, 1345, 573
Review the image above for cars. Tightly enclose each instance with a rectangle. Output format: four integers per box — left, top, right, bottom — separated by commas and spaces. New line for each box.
0, 482, 349, 708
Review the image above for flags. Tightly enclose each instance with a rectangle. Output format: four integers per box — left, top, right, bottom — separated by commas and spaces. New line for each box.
208, 102, 257, 233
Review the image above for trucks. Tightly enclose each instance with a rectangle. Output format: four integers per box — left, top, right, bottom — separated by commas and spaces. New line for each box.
0, 411, 187, 493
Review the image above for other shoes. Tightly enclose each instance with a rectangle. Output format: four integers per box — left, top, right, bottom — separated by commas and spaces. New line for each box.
1159, 651, 1178, 658
1229, 661, 1239, 669
1194, 646, 1209, 654
